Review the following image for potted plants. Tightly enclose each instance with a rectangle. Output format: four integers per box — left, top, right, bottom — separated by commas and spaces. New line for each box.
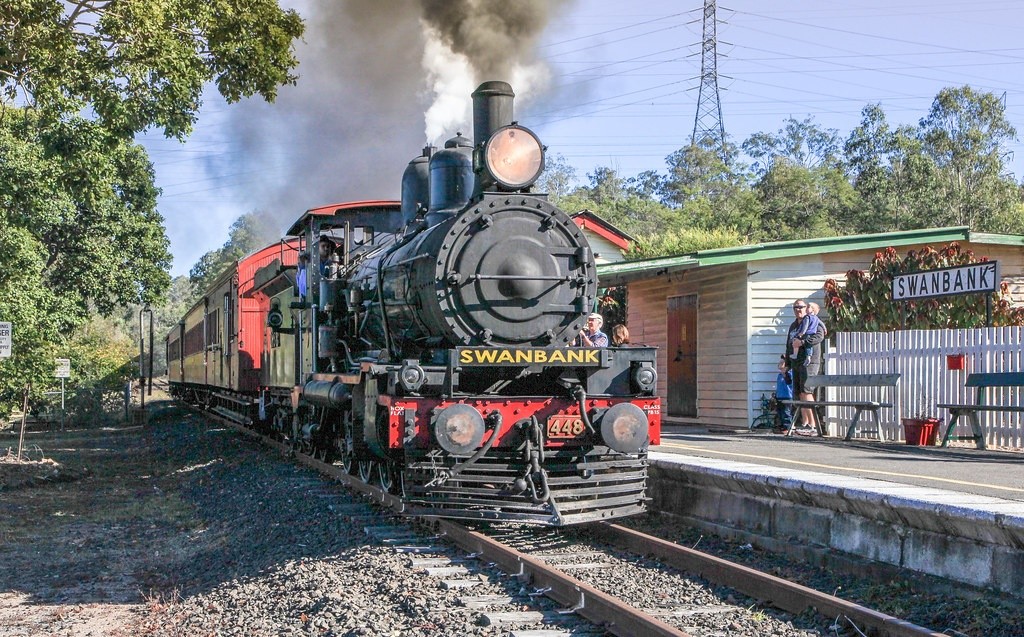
901, 411, 940, 446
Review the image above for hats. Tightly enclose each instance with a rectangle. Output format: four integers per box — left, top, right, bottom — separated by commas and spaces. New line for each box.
588, 312, 603, 325
779, 354, 787, 358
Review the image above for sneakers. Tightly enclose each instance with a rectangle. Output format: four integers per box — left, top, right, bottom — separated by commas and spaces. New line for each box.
795, 423, 820, 438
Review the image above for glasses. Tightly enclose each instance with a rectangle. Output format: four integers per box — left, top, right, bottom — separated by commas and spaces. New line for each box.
793, 306, 807, 309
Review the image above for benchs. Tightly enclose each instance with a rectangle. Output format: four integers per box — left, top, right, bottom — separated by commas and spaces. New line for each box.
937, 371, 1024, 450
7, 421, 56, 431
782, 373, 901, 443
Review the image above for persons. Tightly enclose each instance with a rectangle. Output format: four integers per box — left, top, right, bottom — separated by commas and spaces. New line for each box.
776, 360, 793, 431
299, 235, 336, 297
612, 324, 629, 345
784, 299, 824, 435
789, 302, 827, 366
579, 313, 608, 347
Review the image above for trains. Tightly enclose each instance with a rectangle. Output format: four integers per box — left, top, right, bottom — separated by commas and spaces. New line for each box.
164, 81, 662, 528
